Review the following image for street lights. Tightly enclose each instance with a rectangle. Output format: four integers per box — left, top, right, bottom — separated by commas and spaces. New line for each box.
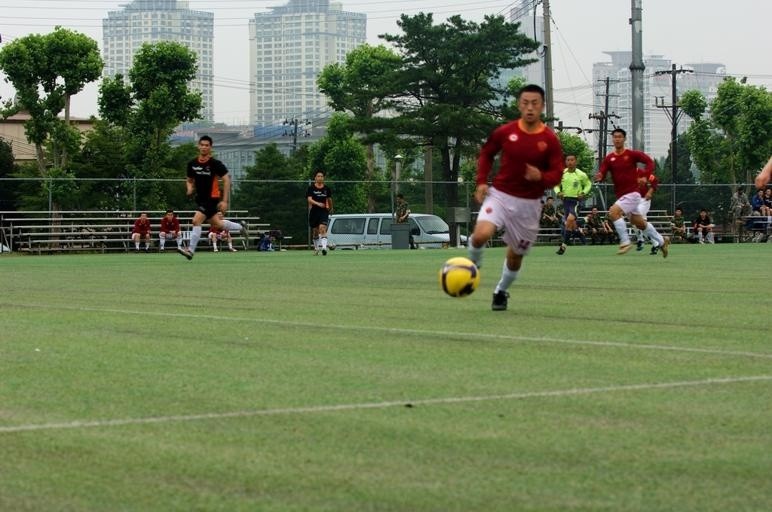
393, 152, 403, 198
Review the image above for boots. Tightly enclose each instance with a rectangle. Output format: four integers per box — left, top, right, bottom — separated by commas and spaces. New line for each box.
590, 238, 621, 245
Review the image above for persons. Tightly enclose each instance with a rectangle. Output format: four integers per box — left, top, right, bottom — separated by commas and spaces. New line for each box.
694, 209, 715, 244
540, 197, 618, 245
159, 210, 183, 251
594, 128, 671, 258
209, 210, 239, 252
176, 135, 248, 260
731, 186, 772, 224
132, 214, 151, 252
396, 194, 416, 249
466, 85, 564, 310
754, 155, 772, 189
307, 170, 333, 255
554, 153, 592, 254
632, 163, 660, 255
670, 209, 686, 243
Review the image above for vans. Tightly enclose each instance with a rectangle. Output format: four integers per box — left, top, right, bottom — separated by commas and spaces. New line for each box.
325, 211, 474, 251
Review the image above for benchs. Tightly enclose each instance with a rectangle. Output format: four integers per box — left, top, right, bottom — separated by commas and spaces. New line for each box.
469, 210, 715, 248
0, 210, 292, 255
728, 205, 772, 243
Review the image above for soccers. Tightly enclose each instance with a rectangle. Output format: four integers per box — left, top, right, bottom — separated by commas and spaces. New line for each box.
437, 257, 480, 297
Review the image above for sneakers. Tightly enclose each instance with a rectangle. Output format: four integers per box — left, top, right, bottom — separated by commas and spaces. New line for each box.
240, 220, 249, 240
698, 239, 713, 244
636, 238, 644, 251
556, 244, 567, 256
649, 244, 660, 255
177, 248, 194, 260
322, 250, 327, 256
661, 237, 670, 258
584, 214, 593, 225
229, 248, 237, 252
213, 249, 219, 252
135, 247, 165, 254
312, 249, 319, 256
492, 290, 509, 311
617, 241, 632, 254
